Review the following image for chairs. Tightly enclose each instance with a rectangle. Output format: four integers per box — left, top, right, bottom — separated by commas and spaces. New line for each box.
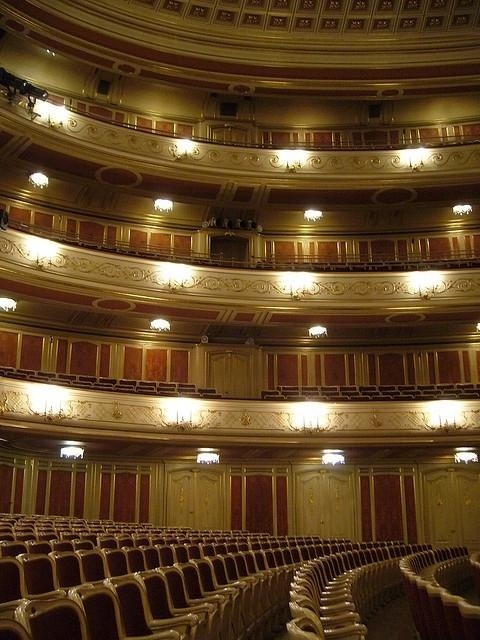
1, 361, 223, 402
1, 513, 480, 639
258, 381, 479, 402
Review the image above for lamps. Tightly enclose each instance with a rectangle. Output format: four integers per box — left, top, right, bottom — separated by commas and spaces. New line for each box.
147, 319, 171, 333
196, 450, 221, 465
303, 209, 323, 222
27, 172, 49, 190
154, 195, 175, 214
321, 452, 348, 467
308, 324, 331, 340
453, 452, 478, 465
452, 204, 473, 216
58, 447, 84, 462
0, 297, 17, 315
